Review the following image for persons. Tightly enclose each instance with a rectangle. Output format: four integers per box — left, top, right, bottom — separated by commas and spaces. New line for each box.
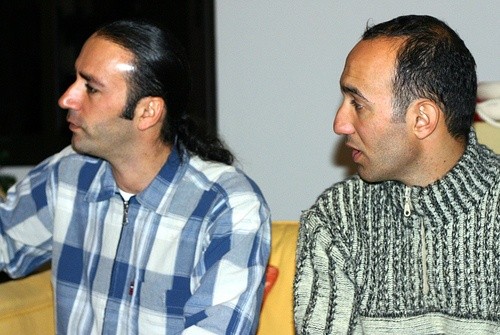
0, 21, 272, 335
292, 15, 500, 335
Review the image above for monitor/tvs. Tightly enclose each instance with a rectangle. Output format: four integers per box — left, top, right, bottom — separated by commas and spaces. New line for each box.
0, 0, 218, 180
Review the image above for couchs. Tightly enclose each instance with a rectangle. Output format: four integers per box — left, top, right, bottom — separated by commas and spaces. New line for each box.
0, 220, 299, 335
